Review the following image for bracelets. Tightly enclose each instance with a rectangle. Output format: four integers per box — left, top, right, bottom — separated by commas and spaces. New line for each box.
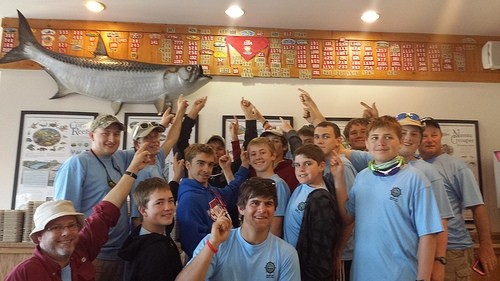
207, 240, 218, 253
125, 171, 137, 179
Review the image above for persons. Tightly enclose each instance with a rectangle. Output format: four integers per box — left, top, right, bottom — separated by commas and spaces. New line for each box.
303, 103, 357, 281
279, 117, 342, 281
360, 102, 498, 281
175, 177, 301, 281
330, 115, 445, 281
340, 112, 455, 281
4, 142, 153, 281
53, 108, 175, 281
118, 151, 185, 281
129, 88, 370, 260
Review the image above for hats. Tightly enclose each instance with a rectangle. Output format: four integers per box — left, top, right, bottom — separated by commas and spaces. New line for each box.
260, 129, 289, 145
397, 113, 426, 130
90, 113, 126, 132
421, 117, 441, 128
29, 200, 84, 245
204, 133, 226, 148
134, 120, 164, 141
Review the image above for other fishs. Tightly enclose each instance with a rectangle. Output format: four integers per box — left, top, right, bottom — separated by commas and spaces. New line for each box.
0, 8, 213, 117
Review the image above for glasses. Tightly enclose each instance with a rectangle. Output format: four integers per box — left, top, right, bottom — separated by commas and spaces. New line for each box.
132, 121, 158, 137
103, 165, 123, 188
44, 222, 81, 235
242, 178, 276, 193
396, 112, 420, 122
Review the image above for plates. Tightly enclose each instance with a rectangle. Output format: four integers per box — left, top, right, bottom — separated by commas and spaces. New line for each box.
0, 200, 46, 243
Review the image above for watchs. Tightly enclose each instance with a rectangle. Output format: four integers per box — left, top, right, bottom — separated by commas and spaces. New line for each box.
435, 256, 447, 265
